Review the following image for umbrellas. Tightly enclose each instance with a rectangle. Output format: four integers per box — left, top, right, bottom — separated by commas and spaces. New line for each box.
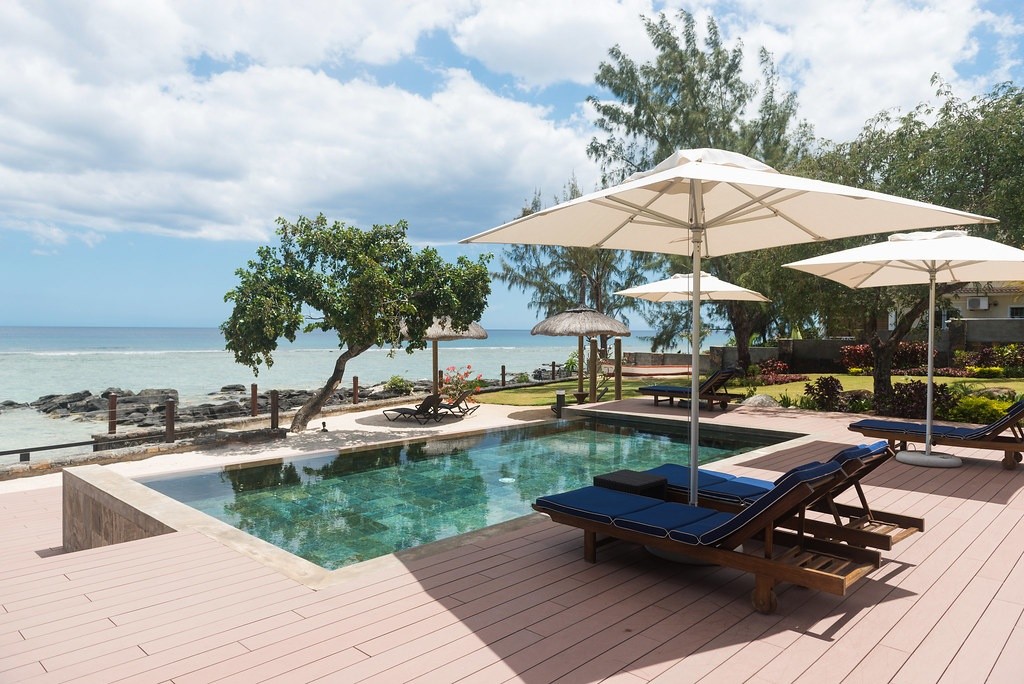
609, 269, 772, 391
396, 315, 489, 396
781, 230, 1024, 470
455, 139, 1000, 505
530, 301, 630, 394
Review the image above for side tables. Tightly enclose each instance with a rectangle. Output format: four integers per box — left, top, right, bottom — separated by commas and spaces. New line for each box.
593, 469, 668, 502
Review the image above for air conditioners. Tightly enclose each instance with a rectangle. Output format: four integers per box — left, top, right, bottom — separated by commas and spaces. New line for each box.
967, 297, 989, 310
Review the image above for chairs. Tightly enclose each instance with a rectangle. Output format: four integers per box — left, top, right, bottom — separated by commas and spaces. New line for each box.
382, 393, 448, 425
531, 461, 882, 616
415, 389, 480, 418
551, 387, 608, 413
847, 399, 1024, 470
639, 370, 733, 411
640, 440, 926, 552
